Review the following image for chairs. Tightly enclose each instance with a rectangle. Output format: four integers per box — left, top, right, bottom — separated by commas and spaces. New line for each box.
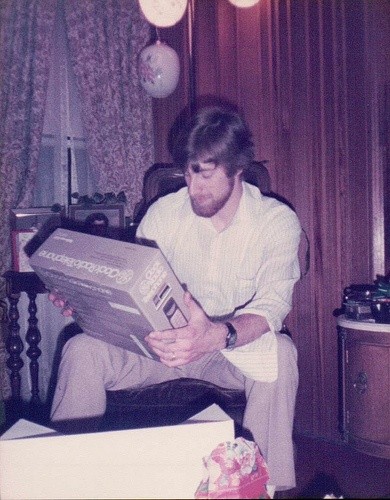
106, 163, 290, 434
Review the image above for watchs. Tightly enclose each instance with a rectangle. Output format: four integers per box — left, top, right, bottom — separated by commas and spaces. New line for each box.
224, 321, 238, 351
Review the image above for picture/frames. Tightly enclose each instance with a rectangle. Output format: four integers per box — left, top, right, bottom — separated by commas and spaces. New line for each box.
10, 206, 66, 230
68, 204, 124, 231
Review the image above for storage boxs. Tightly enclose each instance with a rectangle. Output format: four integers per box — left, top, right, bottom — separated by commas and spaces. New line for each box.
0, 404, 235, 500
28, 228, 191, 371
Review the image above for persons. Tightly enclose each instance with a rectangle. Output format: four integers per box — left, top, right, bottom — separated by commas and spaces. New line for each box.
84, 211, 113, 239
44, 94, 297, 500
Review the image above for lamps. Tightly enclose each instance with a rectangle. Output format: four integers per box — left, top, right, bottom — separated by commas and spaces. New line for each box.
138, 27, 181, 98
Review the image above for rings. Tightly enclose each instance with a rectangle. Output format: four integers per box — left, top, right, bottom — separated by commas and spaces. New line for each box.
171, 351, 176, 362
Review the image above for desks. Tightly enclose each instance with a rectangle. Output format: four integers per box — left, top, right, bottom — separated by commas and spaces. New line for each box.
5, 271, 50, 406
